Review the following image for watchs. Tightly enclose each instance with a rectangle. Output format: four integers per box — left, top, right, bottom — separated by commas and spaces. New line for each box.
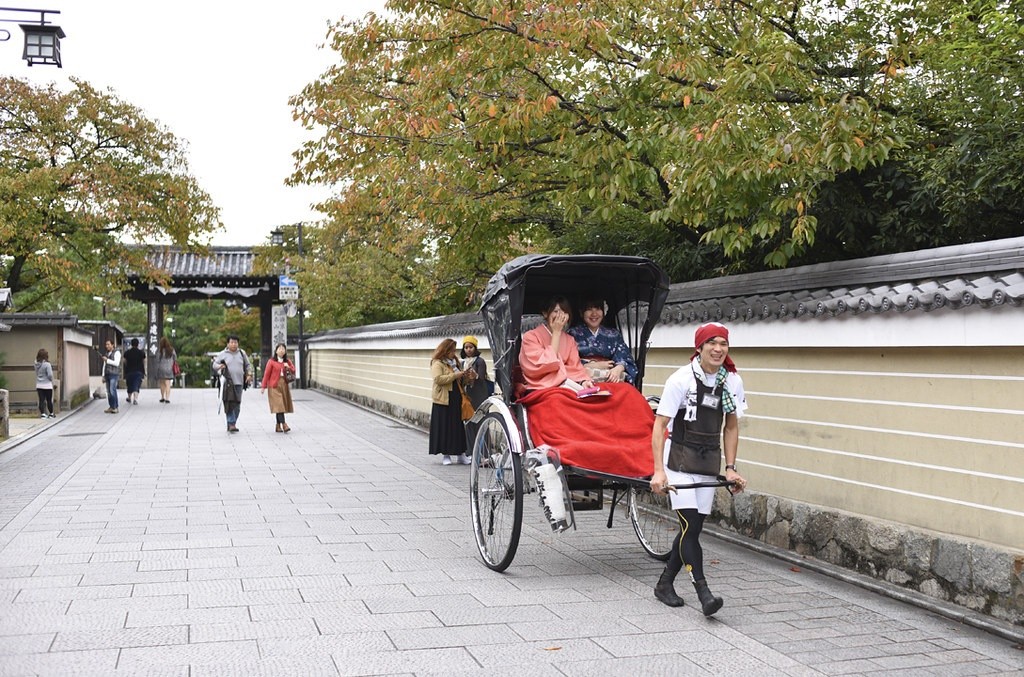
725, 465, 738, 473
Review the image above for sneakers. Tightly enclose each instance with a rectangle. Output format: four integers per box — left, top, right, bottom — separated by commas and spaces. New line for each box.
442, 456, 452, 465
456, 452, 472, 464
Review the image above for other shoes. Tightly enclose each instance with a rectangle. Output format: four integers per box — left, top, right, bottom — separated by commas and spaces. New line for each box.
478, 458, 491, 467
126, 398, 131, 403
133, 400, 137, 405
104, 407, 119, 413
48, 412, 56, 419
40, 413, 48, 419
227, 423, 239, 431
160, 399, 170, 403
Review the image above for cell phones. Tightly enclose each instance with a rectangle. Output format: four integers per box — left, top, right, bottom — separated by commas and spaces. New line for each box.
466, 365, 472, 371
92, 345, 100, 350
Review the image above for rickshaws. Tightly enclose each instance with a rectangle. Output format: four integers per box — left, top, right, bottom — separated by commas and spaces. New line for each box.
468, 252, 749, 619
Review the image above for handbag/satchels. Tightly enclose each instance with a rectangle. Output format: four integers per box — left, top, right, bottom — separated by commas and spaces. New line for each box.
173, 361, 180, 376
466, 378, 495, 400
461, 392, 474, 421
243, 374, 248, 390
222, 378, 240, 403
284, 366, 295, 383
491, 442, 513, 470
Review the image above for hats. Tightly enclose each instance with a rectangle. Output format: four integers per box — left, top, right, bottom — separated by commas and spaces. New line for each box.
463, 335, 478, 347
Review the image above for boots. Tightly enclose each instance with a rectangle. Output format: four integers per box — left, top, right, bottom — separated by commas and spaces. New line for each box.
276, 424, 283, 432
692, 579, 723, 616
282, 422, 290, 433
654, 566, 683, 607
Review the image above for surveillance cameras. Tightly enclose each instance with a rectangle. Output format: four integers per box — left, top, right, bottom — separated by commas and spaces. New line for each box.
92, 295, 106, 303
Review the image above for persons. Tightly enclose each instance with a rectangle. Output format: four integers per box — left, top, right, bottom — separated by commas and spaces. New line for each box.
122, 338, 146, 405
34, 349, 58, 420
458, 336, 493, 467
152, 337, 177, 404
427, 338, 472, 466
261, 342, 296, 433
518, 294, 595, 390
565, 296, 639, 386
184, 356, 219, 389
212, 335, 253, 432
650, 322, 749, 618
101, 340, 123, 414
